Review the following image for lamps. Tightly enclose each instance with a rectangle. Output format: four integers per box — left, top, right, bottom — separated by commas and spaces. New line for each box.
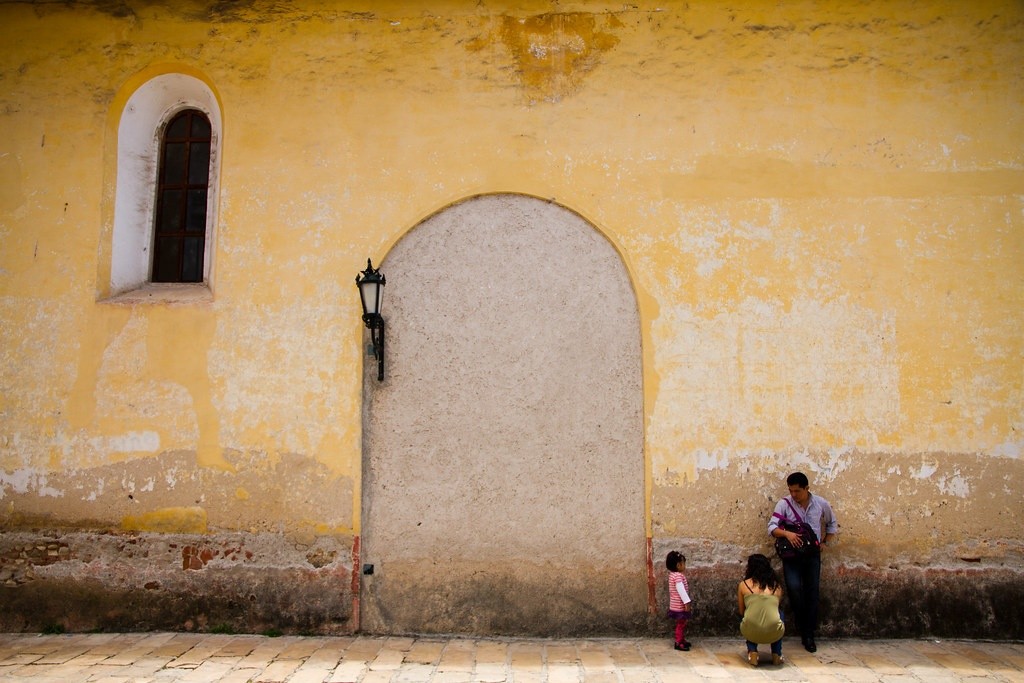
354, 257, 386, 381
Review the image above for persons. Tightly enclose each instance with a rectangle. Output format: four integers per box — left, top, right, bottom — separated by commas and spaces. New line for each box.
738, 554, 785, 666
769, 472, 837, 652
666, 551, 692, 650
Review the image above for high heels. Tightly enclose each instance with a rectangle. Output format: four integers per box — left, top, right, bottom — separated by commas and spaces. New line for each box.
772, 653, 785, 666
747, 651, 759, 666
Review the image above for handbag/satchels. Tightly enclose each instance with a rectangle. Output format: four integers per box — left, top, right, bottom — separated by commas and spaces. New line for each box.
773, 498, 819, 560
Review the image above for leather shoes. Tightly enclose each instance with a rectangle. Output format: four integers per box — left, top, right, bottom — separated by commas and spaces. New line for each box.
802, 635, 816, 652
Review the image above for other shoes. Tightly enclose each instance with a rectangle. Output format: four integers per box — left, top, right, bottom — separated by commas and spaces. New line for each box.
683, 639, 691, 647
675, 642, 689, 650
